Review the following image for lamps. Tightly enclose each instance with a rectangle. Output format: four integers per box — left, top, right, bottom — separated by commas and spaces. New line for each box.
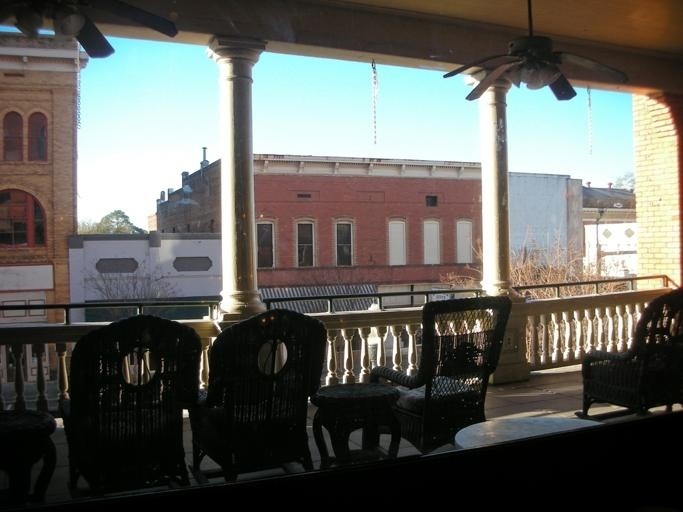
12, 0, 85, 40
504, 60, 563, 91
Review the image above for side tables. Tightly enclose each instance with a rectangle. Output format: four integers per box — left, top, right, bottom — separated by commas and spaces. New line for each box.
0, 409, 58, 509
310, 384, 399, 473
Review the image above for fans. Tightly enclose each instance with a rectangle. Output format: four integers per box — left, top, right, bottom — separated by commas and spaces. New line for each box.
443, 0, 629, 100
0, 0, 179, 57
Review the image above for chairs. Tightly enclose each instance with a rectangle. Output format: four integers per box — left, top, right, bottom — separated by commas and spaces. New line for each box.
186, 308, 327, 486
575, 287, 683, 422
373, 297, 512, 456
60, 314, 202, 499
